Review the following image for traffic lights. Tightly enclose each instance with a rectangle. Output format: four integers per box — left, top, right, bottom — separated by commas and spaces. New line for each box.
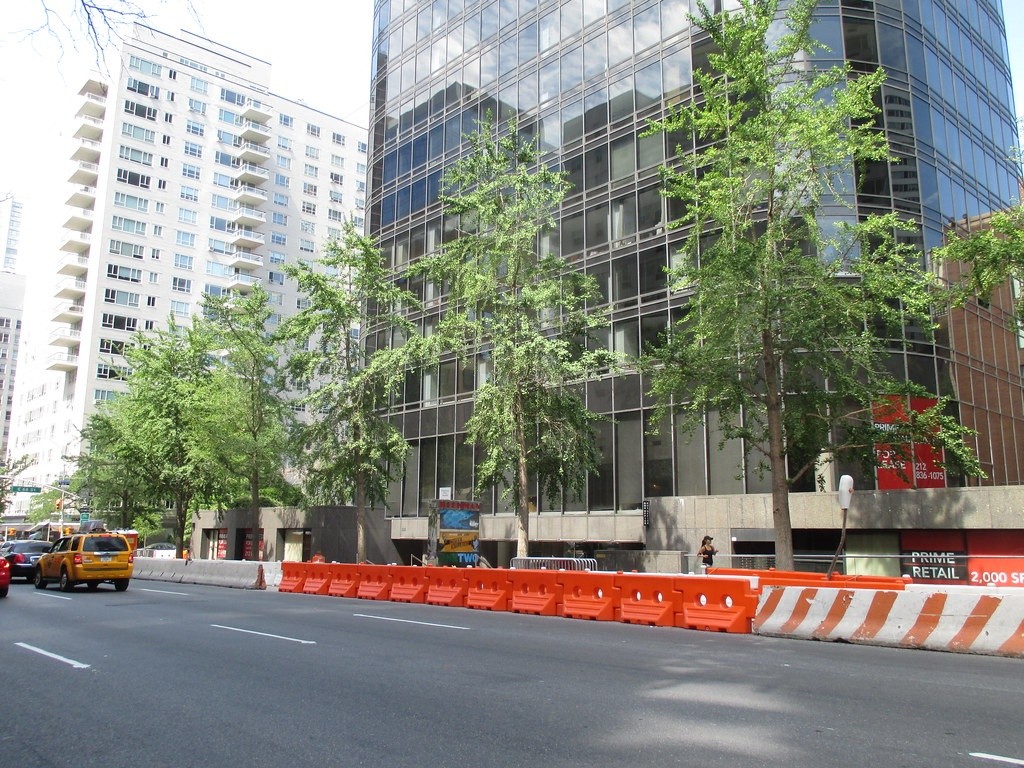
56, 501, 62, 510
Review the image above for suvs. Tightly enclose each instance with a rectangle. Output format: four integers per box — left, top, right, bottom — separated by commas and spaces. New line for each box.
33, 530, 134, 592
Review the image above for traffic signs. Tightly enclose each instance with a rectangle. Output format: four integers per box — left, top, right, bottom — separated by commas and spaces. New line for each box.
11, 486, 42, 493
81, 513, 89, 519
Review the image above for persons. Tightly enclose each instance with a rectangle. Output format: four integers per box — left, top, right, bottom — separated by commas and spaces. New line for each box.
697, 536, 719, 575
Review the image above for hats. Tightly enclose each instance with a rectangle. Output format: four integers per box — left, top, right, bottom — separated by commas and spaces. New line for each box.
704, 536, 713, 540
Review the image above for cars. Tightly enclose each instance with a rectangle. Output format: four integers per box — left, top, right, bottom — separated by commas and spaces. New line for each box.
141, 542, 177, 551
0, 540, 54, 598
